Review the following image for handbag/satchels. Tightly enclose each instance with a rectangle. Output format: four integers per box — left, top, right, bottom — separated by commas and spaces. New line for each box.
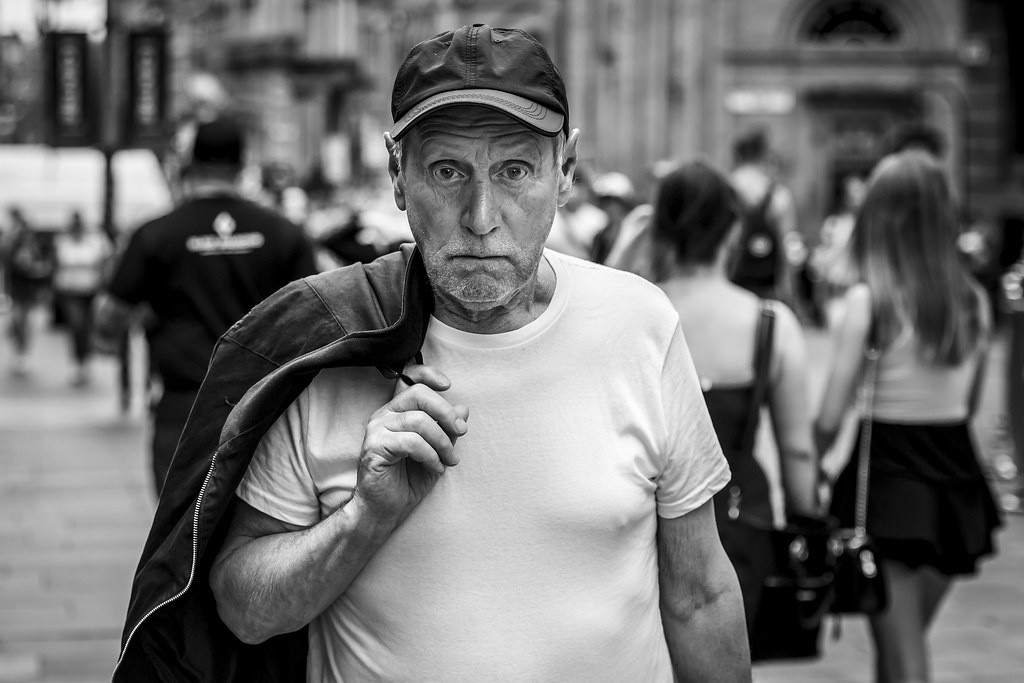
818, 536, 892, 617
715, 515, 824, 665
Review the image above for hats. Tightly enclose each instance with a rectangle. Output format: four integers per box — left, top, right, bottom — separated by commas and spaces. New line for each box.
391, 23, 565, 138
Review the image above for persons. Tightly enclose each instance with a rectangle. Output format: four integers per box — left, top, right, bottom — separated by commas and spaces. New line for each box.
651, 146, 1008, 683
0, 119, 1024, 516
110, 23, 753, 683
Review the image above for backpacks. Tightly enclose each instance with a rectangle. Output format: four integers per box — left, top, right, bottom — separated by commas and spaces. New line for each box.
726, 181, 783, 290
